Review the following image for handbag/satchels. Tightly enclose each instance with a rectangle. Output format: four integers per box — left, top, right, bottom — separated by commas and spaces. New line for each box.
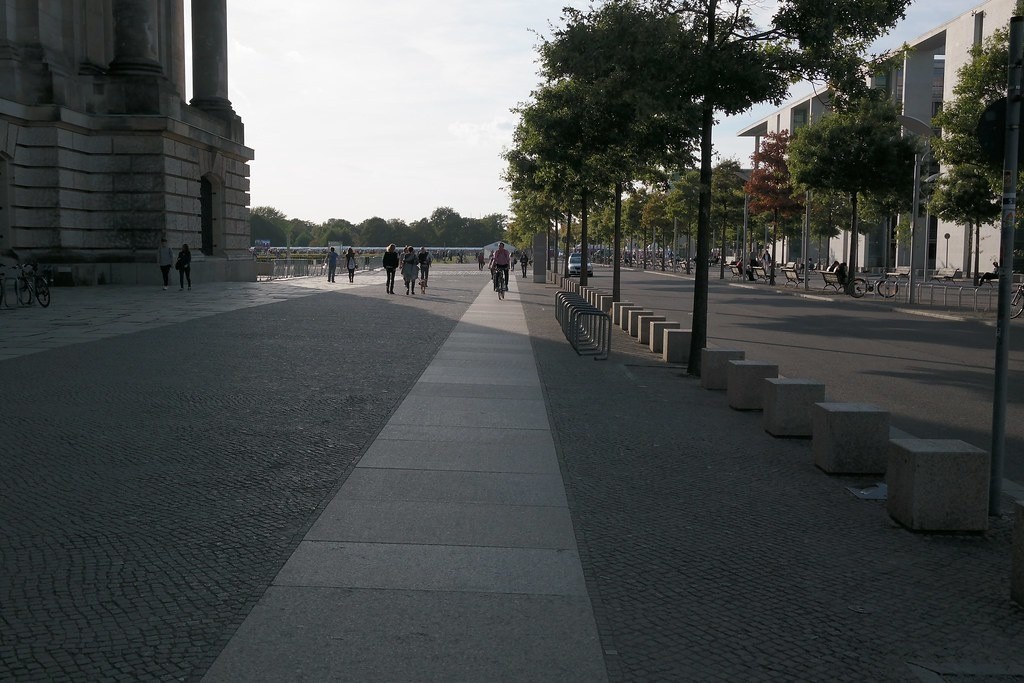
176, 260, 182, 271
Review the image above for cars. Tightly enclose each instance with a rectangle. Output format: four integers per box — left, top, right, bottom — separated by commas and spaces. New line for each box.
568, 253, 593, 277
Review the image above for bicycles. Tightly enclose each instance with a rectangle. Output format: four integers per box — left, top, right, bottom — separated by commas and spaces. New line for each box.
11, 263, 51, 308
0, 264, 6, 308
848, 267, 898, 298
488, 268, 510, 300
1010, 283, 1024, 319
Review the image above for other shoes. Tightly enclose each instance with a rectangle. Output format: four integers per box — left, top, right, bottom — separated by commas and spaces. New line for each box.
505, 285, 508, 291
180, 288, 183, 291
390, 291, 394, 294
407, 290, 409, 295
387, 290, 389, 294
163, 286, 167, 290
188, 287, 191, 291
412, 291, 415, 294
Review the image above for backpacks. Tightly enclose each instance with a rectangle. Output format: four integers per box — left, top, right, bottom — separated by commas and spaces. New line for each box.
348, 256, 355, 270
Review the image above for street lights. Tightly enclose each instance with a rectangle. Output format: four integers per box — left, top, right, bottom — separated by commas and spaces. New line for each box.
895, 114, 938, 304
923, 172, 945, 282
734, 172, 751, 281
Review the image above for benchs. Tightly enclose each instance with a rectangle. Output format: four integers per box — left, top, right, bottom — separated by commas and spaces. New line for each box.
927, 267, 960, 284
728, 266, 749, 281
817, 271, 845, 294
781, 261, 810, 288
753, 266, 776, 285
887, 265, 911, 285
666, 261, 694, 275
978, 271, 1014, 287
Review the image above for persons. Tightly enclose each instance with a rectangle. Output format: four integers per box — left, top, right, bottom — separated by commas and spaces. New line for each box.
489, 242, 511, 291
736, 247, 771, 281
436, 249, 465, 264
509, 252, 517, 270
177, 243, 192, 292
383, 244, 399, 294
520, 252, 528, 278
399, 247, 409, 287
529, 254, 534, 266
345, 248, 359, 283
714, 256, 719, 263
327, 247, 337, 282
979, 262, 1000, 286
157, 239, 174, 290
418, 246, 434, 287
798, 255, 814, 274
477, 252, 484, 270
675, 254, 682, 264
624, 251, 630, 266
401, 246, 419, 295
827, 260, 848, 294
